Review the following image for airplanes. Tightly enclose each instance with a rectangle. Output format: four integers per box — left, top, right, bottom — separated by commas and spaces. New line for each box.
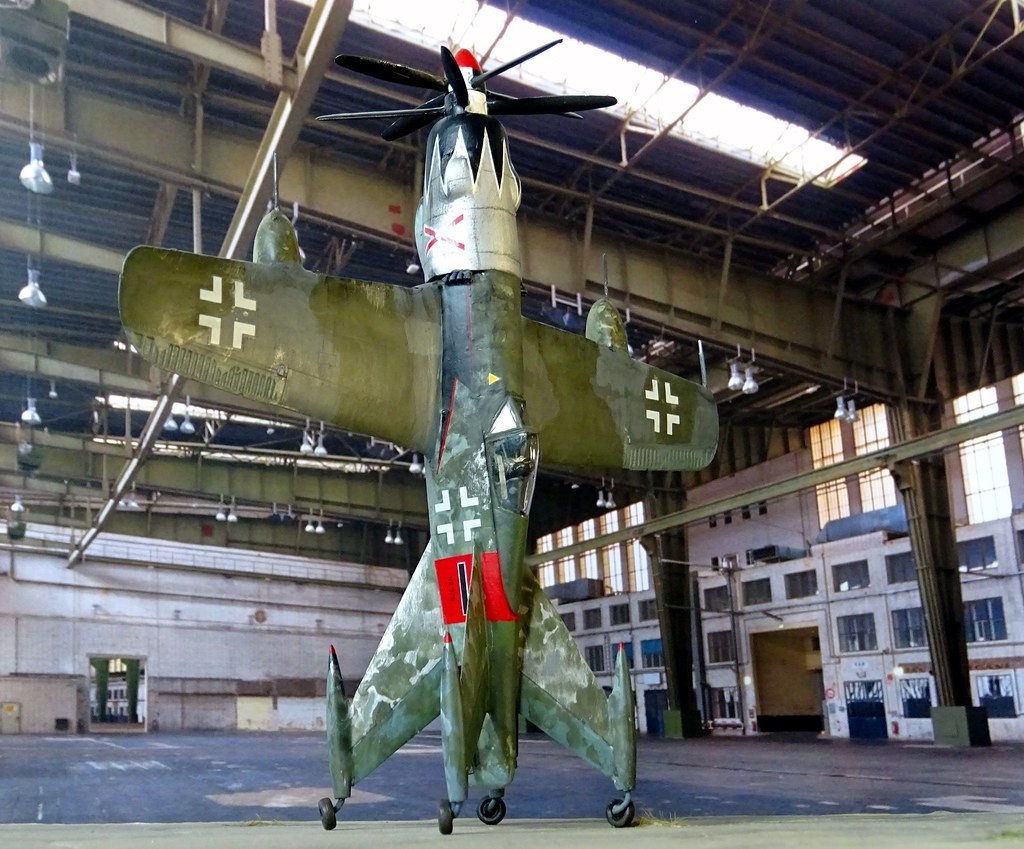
119, 39, 720, 834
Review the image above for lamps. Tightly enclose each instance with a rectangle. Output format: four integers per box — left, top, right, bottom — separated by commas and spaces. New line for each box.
216, 508, 226, 520
10, 494, 25, 513
21, 398, 42, 424
179, 412, 195, 434
834, 397, 850, 419
300, 432, 313, 453
163, 412, 177, 430
394, 531, 404, 544
726, 362, 746, 391
18, 267, 46, 308
409, 455, 422, 473
304, 521, 314, 534
384, 530, 394, 543
315, 522, 325, 534
18, 143, 55, 195
314, 437, 328, 456
846, 400, 861, 423
228, 510, 238, 523
742, 368, 758, 395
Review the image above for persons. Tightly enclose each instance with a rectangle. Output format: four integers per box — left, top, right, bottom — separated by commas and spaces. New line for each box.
493, 454, 535, 478
